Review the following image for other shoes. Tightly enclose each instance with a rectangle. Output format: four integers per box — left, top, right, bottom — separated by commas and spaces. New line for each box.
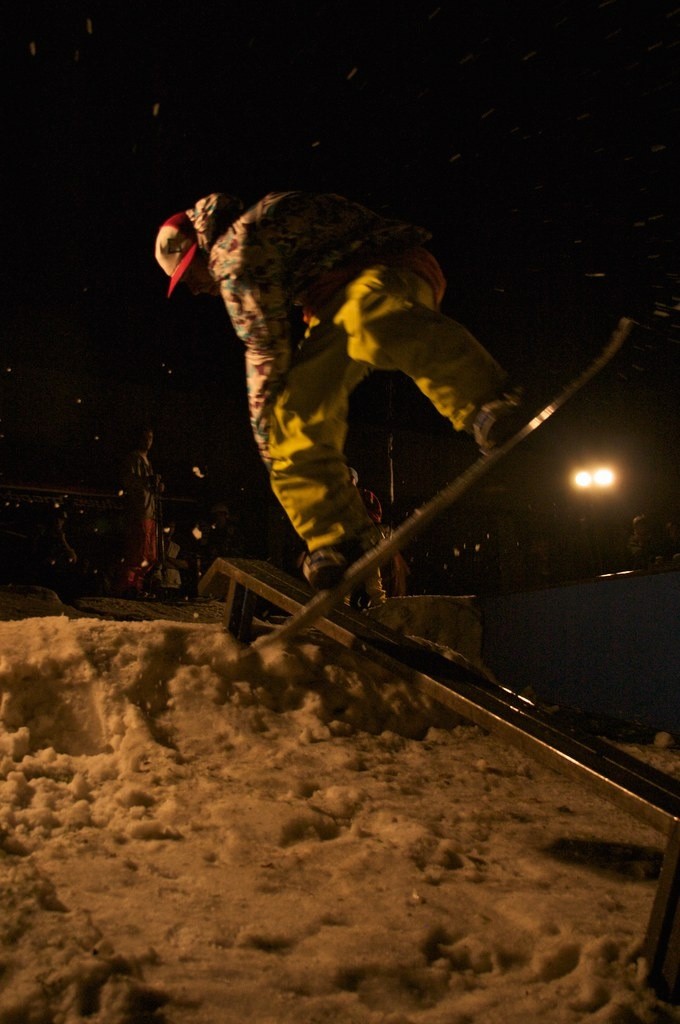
473, 393, 531, 454
122, 589, 156, 601
302, 540, 361, 589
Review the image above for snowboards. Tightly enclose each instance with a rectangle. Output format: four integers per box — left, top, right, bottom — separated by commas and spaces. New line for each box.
248, 314, 637, 654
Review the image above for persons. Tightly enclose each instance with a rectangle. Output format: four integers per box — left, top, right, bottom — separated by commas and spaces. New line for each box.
41, 427, 680, 614
155, 193, 521, 586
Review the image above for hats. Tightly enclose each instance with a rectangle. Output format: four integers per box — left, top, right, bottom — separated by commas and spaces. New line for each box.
154, 212, 198, 297
209, 505, 229, 514
